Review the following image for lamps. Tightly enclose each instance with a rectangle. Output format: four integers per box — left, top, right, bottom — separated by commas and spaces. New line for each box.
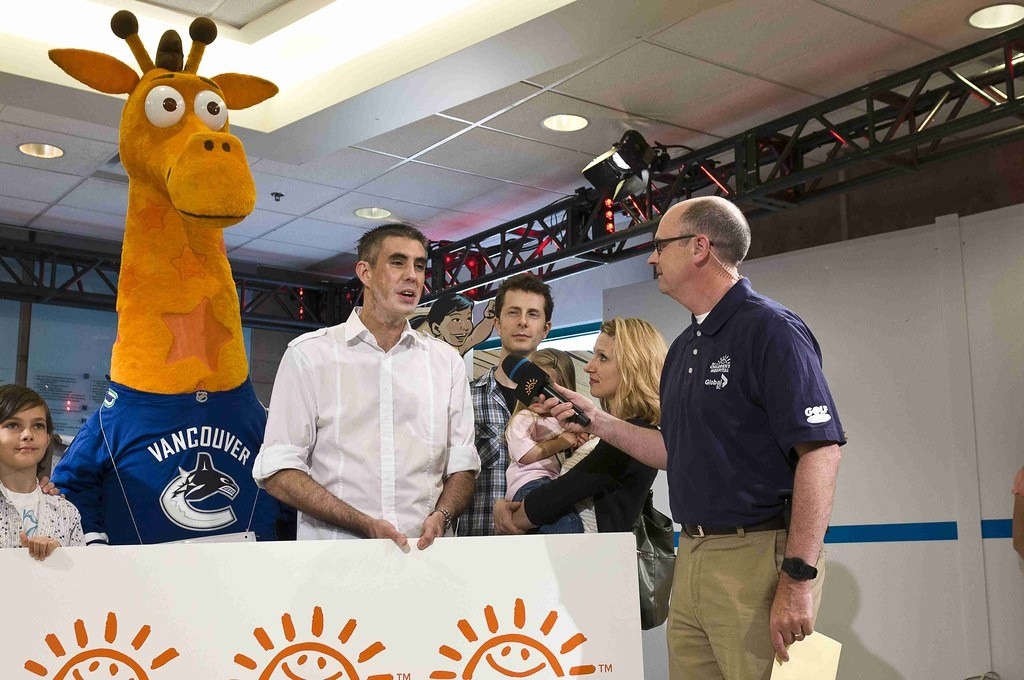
582, 147, 634, 189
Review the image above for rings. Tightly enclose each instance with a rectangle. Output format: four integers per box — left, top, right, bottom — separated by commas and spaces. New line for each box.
795, 633, 801, 636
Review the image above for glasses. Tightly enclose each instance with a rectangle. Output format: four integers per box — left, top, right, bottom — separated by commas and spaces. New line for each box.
653, 235, 714, 256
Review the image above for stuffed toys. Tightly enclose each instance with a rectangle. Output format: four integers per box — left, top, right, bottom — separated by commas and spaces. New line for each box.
40, 10, 295, 545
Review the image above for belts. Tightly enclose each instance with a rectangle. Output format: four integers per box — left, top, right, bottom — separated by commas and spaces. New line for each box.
680, 518, 786, 538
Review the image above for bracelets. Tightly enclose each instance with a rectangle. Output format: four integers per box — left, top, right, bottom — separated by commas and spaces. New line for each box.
435, 508, 451, 533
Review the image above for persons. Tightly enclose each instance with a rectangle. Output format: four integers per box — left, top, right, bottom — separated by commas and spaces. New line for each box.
456, 273, 556, 536
1012, 466, 1024, 556
251, 224, 481, 549
0, 384, 85, 560
492, 317, 667, 535
541, 196, 847, 680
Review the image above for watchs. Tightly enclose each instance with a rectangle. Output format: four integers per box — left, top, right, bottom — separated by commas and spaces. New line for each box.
781, 557, 817, 579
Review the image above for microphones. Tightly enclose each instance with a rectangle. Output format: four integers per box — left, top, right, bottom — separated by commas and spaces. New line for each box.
502, 354, 591, 428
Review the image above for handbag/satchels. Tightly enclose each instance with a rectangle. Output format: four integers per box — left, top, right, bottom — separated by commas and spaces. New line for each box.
633, 489, 676, 630
